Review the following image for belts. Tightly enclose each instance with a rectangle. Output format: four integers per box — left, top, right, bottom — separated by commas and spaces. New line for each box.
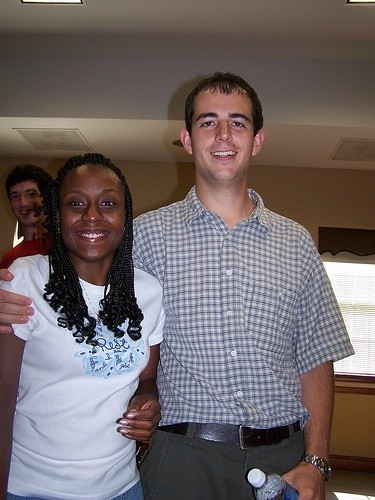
158, 422, 301, 446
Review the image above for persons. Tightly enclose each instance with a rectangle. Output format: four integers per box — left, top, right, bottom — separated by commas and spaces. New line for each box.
0, 153, 166, 500
0, 164, 55, 270
0, 72, 355, 500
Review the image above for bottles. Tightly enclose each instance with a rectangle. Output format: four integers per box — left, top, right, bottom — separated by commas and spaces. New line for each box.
247, 468, 299, 500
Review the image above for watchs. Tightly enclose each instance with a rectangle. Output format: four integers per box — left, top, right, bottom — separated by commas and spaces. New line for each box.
302, 453, 334, 481
